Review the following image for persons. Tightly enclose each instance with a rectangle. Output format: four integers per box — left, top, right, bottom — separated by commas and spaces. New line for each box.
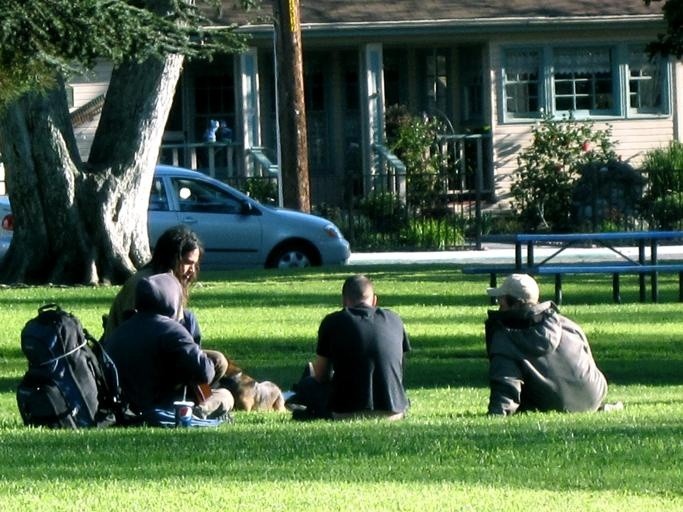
218, 122, 232, 142
484, 272, 624, 416
100, 223, 232, 427
204, 120, 220, 142
288, 274, 411, 423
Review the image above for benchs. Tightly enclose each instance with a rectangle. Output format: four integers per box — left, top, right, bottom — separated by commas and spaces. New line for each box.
515, 263, 682, 302
463, 261, 681, 305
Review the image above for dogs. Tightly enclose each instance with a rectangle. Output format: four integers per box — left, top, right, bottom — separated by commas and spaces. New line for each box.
219, 361, 286, 413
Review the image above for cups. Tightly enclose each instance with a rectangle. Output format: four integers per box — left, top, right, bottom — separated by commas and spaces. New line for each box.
172, 400, 195, 428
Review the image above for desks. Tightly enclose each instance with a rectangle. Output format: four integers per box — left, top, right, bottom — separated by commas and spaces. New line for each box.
471, 231, 683, 305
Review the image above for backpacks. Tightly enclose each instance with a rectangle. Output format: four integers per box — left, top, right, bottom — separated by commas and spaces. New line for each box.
17, 305, 135, 428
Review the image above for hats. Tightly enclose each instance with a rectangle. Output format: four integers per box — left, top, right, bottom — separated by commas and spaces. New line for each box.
487, 274, 539, 304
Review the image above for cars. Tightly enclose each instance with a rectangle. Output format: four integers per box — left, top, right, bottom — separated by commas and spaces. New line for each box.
0, 165, 355, 273
